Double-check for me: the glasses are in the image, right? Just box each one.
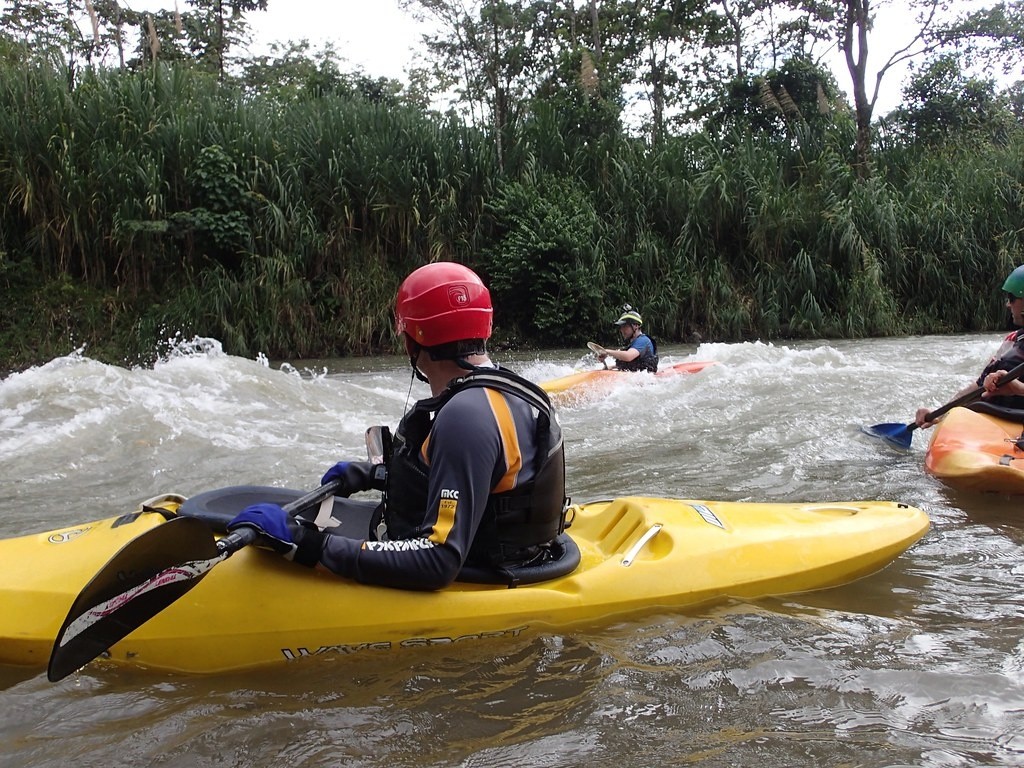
[1007,292,1024,303]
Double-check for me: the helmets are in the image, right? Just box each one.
[395,262,493,346]
[614,303,643,327]
[1001,265,1024,298]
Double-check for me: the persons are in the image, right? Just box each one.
[598,311,660,376]
[223,262,570,592]
[915,263,1024,429]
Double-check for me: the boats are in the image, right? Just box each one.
[538,362,716,397]
[926,381,1024,497]
[0,496,934,673]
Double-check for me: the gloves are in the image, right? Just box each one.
[321,461,377,499]
[226,502,321,567]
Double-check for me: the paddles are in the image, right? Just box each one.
[870,359,1023,448]
[45,457,375,685]
[586,341,609,369]
